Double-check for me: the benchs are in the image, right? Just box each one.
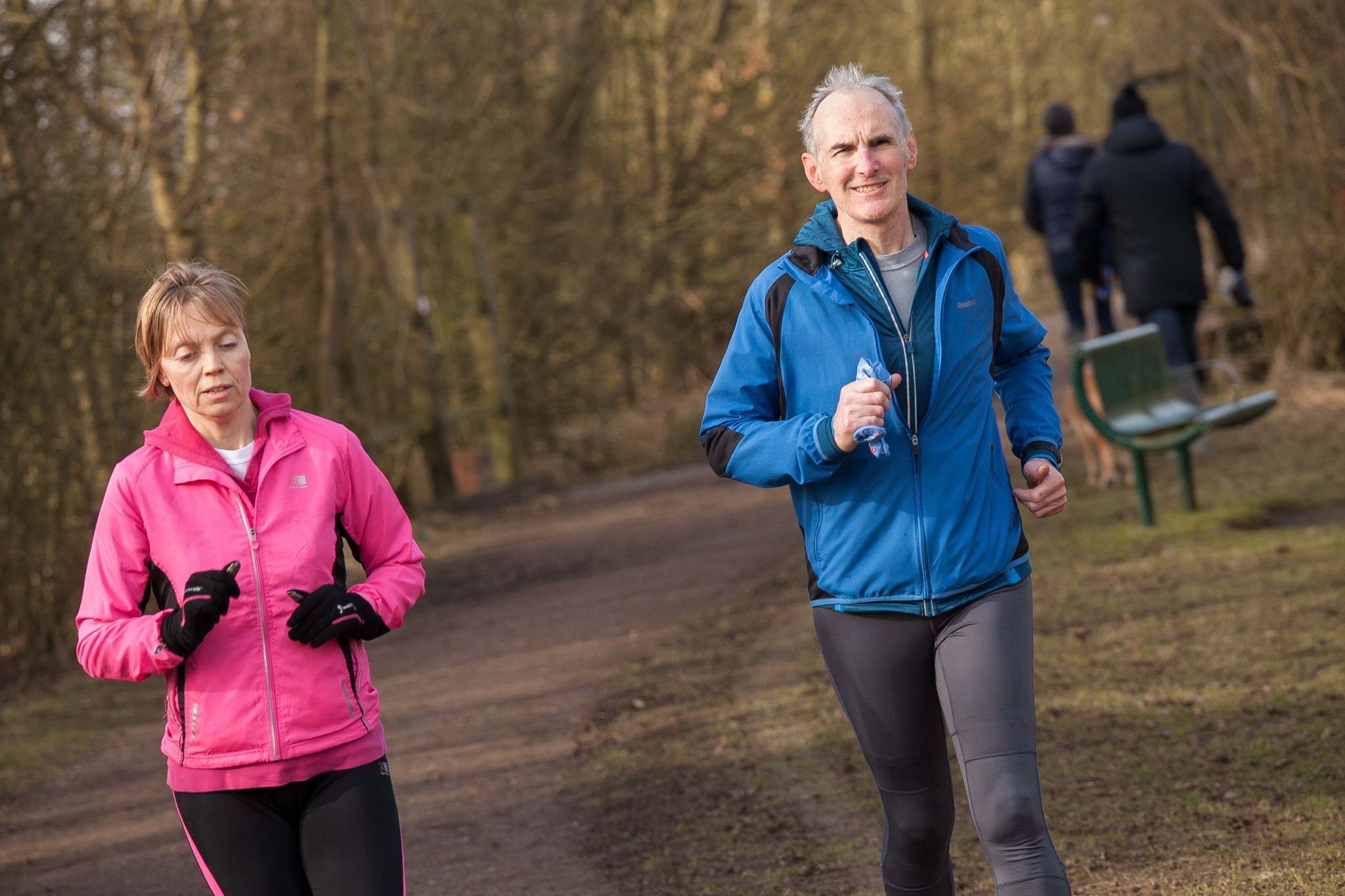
[1068,323,1278,530]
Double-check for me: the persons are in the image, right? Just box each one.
[1020,106,1117,353]
[72,267,429,896]
[698,63,1076,896]
[1085,84,1252,398]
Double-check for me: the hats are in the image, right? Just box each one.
[1114,84,1149,123]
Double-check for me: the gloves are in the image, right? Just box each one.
[168,568,240,652]
[284,584,391,649]
